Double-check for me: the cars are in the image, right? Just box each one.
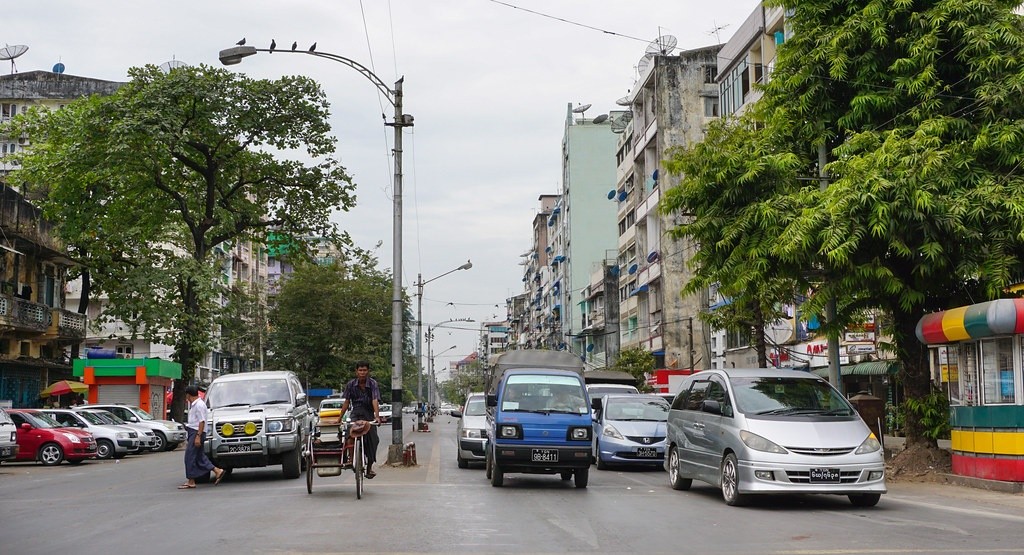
[661,366,889,508]
[590,393,673,472]
[378,402,392,425]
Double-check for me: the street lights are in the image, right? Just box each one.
[218,45,414,468]
[414,260,476,432]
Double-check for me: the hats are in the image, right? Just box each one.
[349,420,371,438]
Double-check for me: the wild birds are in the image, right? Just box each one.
[292,41,297,50]
[309,42,317,52]
[270,39,276,53]
[235,37,246,46]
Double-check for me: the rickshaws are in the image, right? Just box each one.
[303,406,380,500]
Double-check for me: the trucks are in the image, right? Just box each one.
[483,348,602,488]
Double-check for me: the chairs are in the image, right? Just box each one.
[521,384,552,409]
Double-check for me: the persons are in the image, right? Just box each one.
[431,404,436,416]
[459,405,463,415]
[546,385,585,412]
[79,393,88,405]
[178,385,226,489]
[44,397,52,408]
[507,384,527,410]
[422,402,426,418]
[329,361,382,480]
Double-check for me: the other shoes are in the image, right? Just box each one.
[366,471,376,479]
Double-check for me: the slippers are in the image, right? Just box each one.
[178,485,197,489]
[214,470,226,486]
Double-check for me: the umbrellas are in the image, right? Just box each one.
[39,380,89,397]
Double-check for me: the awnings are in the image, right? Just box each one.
[810,361,895,378]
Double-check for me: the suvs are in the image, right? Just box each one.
[200,369,315,480]
[0,407,21,466]
[70,401,188,453]
[4,408,100,466]
[34,407,158,460]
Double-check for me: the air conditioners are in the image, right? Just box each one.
[115,353,133,359]
[17,137,30,146]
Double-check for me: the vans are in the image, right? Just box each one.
[313,398,351,430]
[450,391,491,469]
[581,383,640,426]
